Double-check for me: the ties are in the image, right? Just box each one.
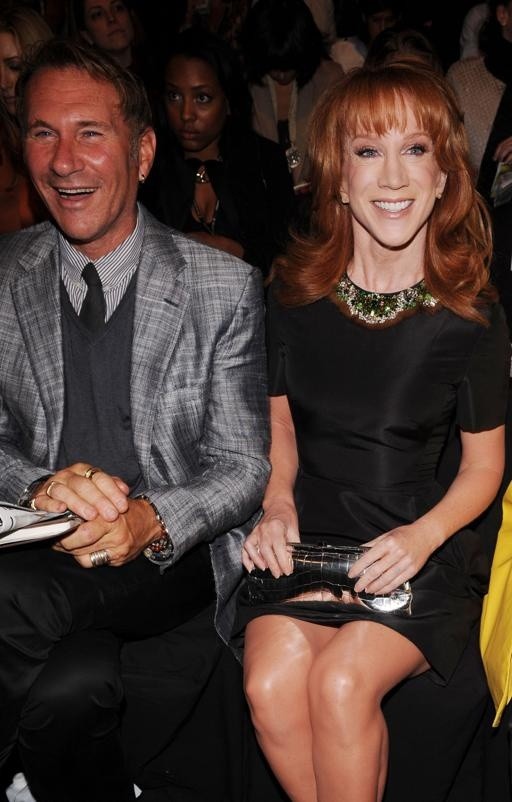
[78,262,106,336]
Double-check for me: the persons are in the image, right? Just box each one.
[0,30,276,800]
[229,49,509,800]
[0,0,512,323]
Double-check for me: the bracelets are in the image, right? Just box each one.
[134,493,170,553]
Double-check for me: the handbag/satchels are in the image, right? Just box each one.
[245,542,414,628]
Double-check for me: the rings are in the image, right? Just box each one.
[83,466,105,480]
[90,548,111,568]
[46,481,57,496]
[31,498,37,511]
